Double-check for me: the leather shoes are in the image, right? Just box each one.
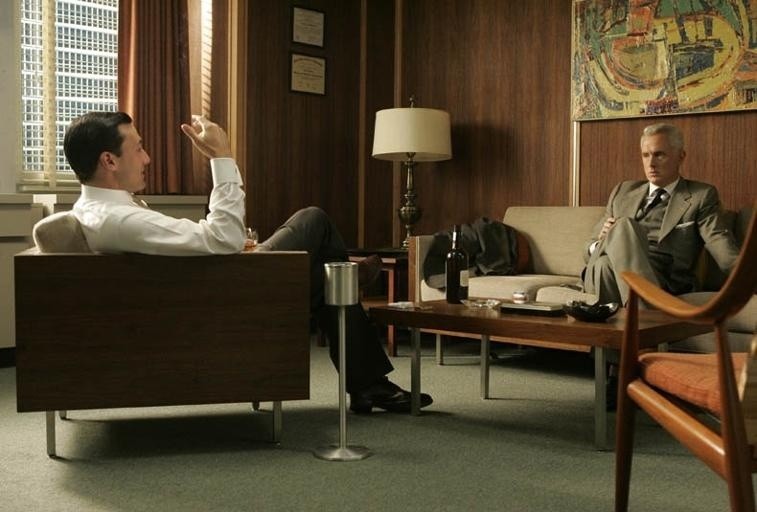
[350,385,433,413]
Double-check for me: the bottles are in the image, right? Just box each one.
[446,225,469,303]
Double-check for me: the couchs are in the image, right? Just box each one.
[12,210,311,457]
[405,205,757,366]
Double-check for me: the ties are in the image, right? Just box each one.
[635,189,666,220]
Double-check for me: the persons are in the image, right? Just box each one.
[65,111,434,415]
[582,124,744,405]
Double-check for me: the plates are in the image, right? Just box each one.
[460,298,501,310]
[562,301,619,322]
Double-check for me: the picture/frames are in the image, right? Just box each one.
[290,52,327,95]
[571,0,757,122]
[292,5,326,48]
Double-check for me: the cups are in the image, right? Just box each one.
[512,291,529,305]
[245,227,257,252]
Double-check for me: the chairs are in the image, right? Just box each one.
[611,208,756,512]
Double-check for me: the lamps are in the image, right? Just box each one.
[372,93,452,247]
